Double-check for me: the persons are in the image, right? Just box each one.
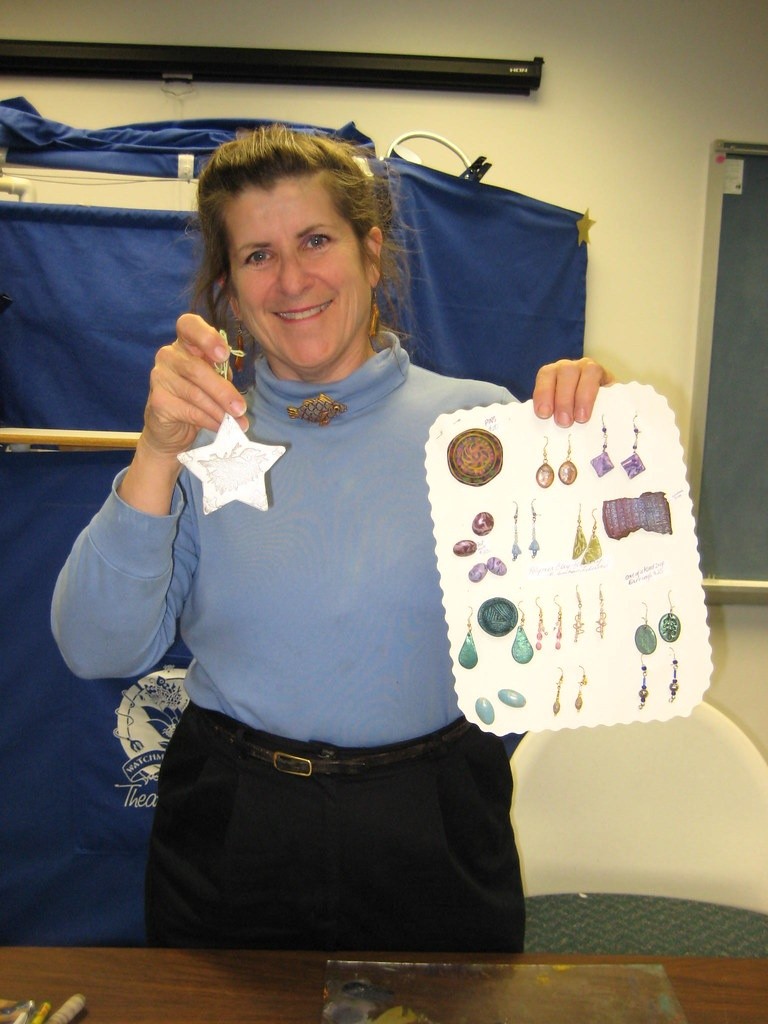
[52,128,608,961]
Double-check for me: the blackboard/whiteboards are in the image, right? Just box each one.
[687,138,768,608]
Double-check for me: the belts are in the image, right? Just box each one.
[192,707,472,777]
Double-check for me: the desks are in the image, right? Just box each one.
[1,946,768,1023]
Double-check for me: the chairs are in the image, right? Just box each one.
[508,701,768,958]
